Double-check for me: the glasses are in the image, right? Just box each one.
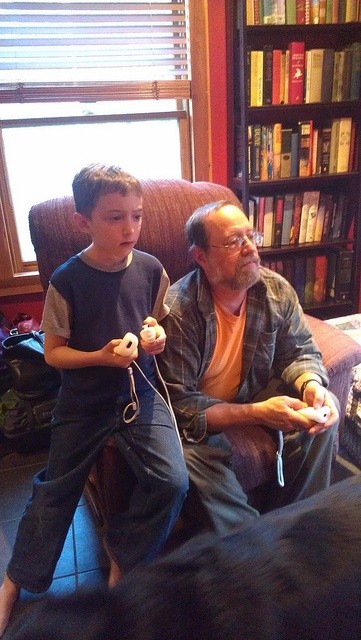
[198,233,264,254]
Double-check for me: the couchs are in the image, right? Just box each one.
[325,309,361,473]
[27,178,360,549]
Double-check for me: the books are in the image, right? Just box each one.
[250,190,359,252]
[244,38,361,115]
[248,118,361,190]
[261,254,353,309]
[248,0,361,28]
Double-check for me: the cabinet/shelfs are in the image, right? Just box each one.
[227,0,361,322]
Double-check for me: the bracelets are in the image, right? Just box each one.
[299,379,320,402]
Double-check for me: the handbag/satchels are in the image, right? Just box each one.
[1,312,40,336]
[0,329,61,391]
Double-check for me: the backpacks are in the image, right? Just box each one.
[0,388,60,453]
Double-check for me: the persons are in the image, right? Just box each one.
[160,199,343,529]
[0,165,190,640]
